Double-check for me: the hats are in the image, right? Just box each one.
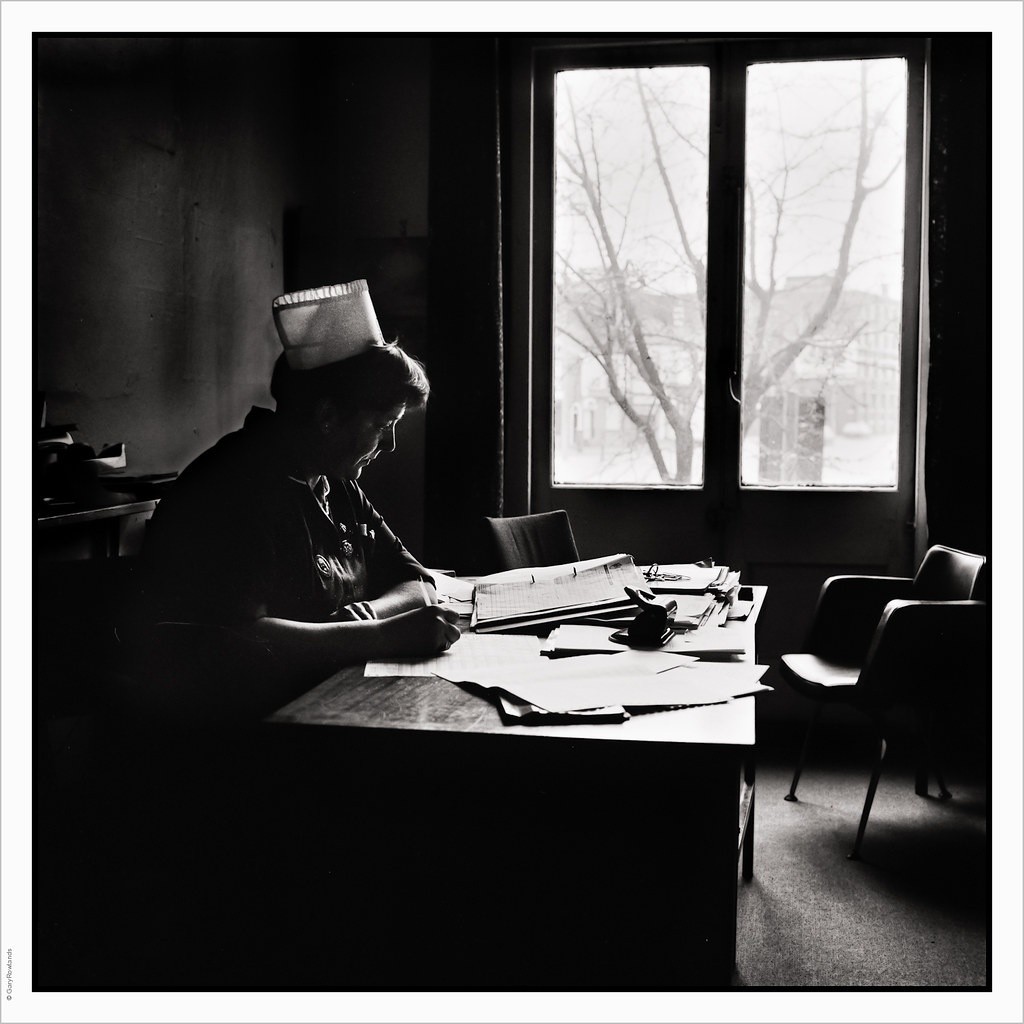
[271,278,384,371]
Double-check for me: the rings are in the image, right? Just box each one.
[444,641,450,649]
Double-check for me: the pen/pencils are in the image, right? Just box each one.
[415,572,431,606]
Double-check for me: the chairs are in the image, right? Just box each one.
[480,509,581,575]
[33,501,277,949]
[779,544,992,861]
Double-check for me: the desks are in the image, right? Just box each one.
[263,586,758,993]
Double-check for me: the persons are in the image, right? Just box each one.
[108,278,459,720]
